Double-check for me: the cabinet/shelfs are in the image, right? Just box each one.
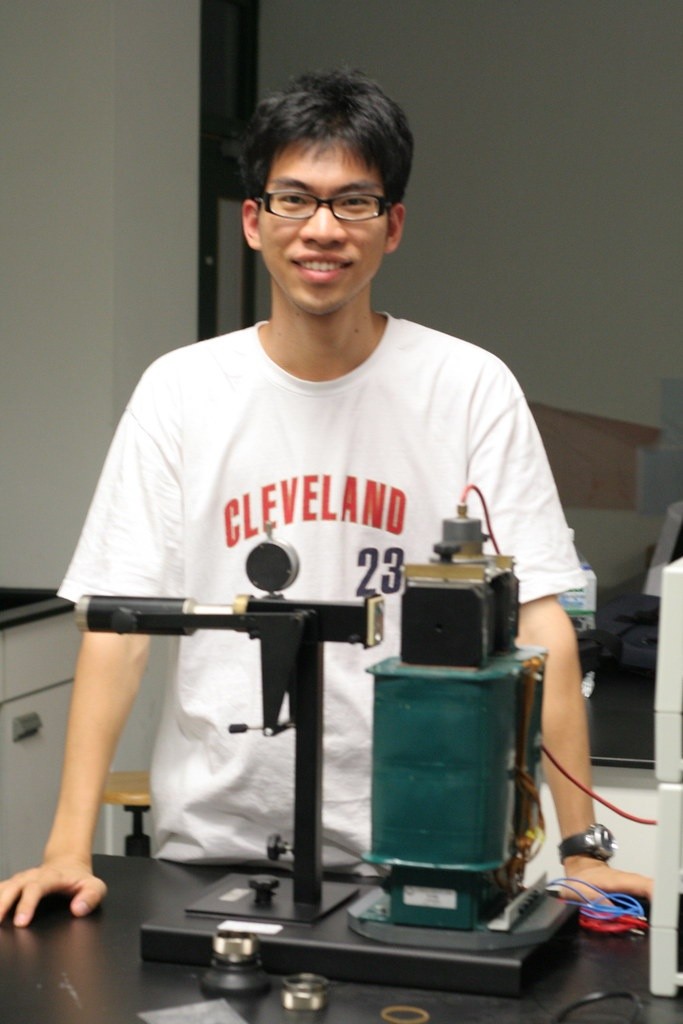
[0,612,116,879]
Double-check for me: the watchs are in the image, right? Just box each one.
[559,822,616,866]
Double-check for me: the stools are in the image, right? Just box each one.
[101,770,153,858]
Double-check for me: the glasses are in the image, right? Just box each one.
[261,191,387,222]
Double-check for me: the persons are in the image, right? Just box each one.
[0,72,654,927]
